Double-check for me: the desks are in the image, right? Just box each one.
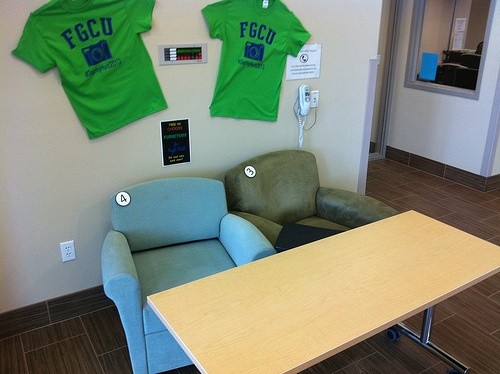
[146,209,500,374]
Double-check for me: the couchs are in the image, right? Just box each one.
[100,177,277,374]
[224,150,400,253]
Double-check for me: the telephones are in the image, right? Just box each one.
[296,85,311,150]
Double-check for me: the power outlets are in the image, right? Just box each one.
[310,90,319,108]
[59,240,76,263]
